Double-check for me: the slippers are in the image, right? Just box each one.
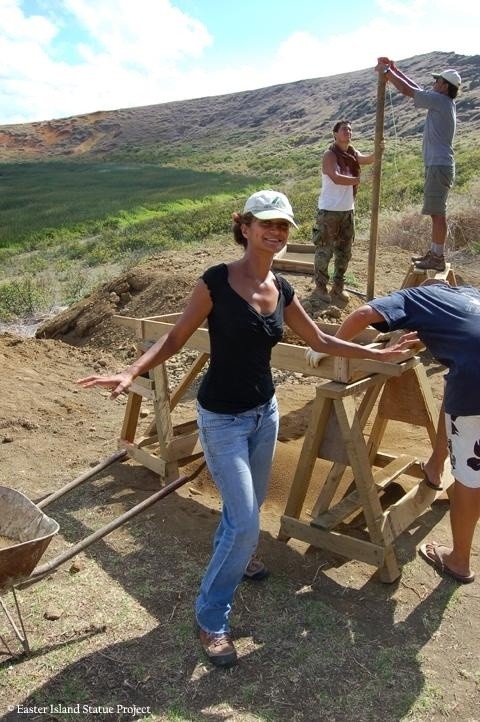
[420,543,475,582]
[419,460,443,491]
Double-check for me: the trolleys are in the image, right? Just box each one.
[0,448,190,661]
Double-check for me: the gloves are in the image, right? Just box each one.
[303,347,330,368]
[373,330,404,349]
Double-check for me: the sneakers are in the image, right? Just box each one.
[199,625,237,668]
[411,249,430,263]
[244,555,267,579]
[414,251,446,271]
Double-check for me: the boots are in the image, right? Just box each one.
[331,278,349,302]
[313,279,332,302]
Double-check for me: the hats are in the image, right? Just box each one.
[431,68,462,90]
[242,189,300,231]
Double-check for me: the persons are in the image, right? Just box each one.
[304,278,480,583]
[312,121,386,300]
[374,59,461,272]
[76,190,420,666]
